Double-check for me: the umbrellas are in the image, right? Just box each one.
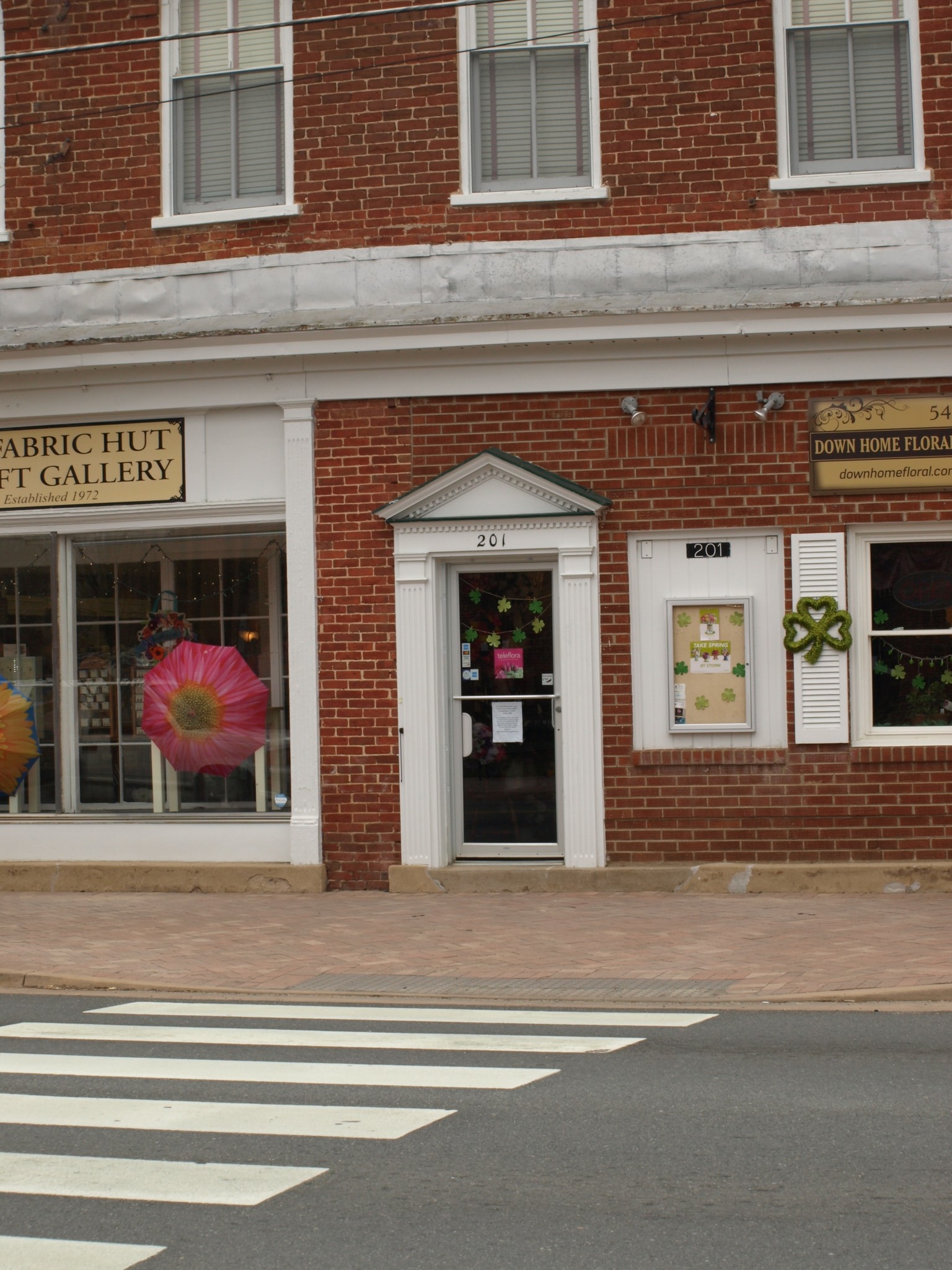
[140,639,269,778]
[0,677,43,798]
